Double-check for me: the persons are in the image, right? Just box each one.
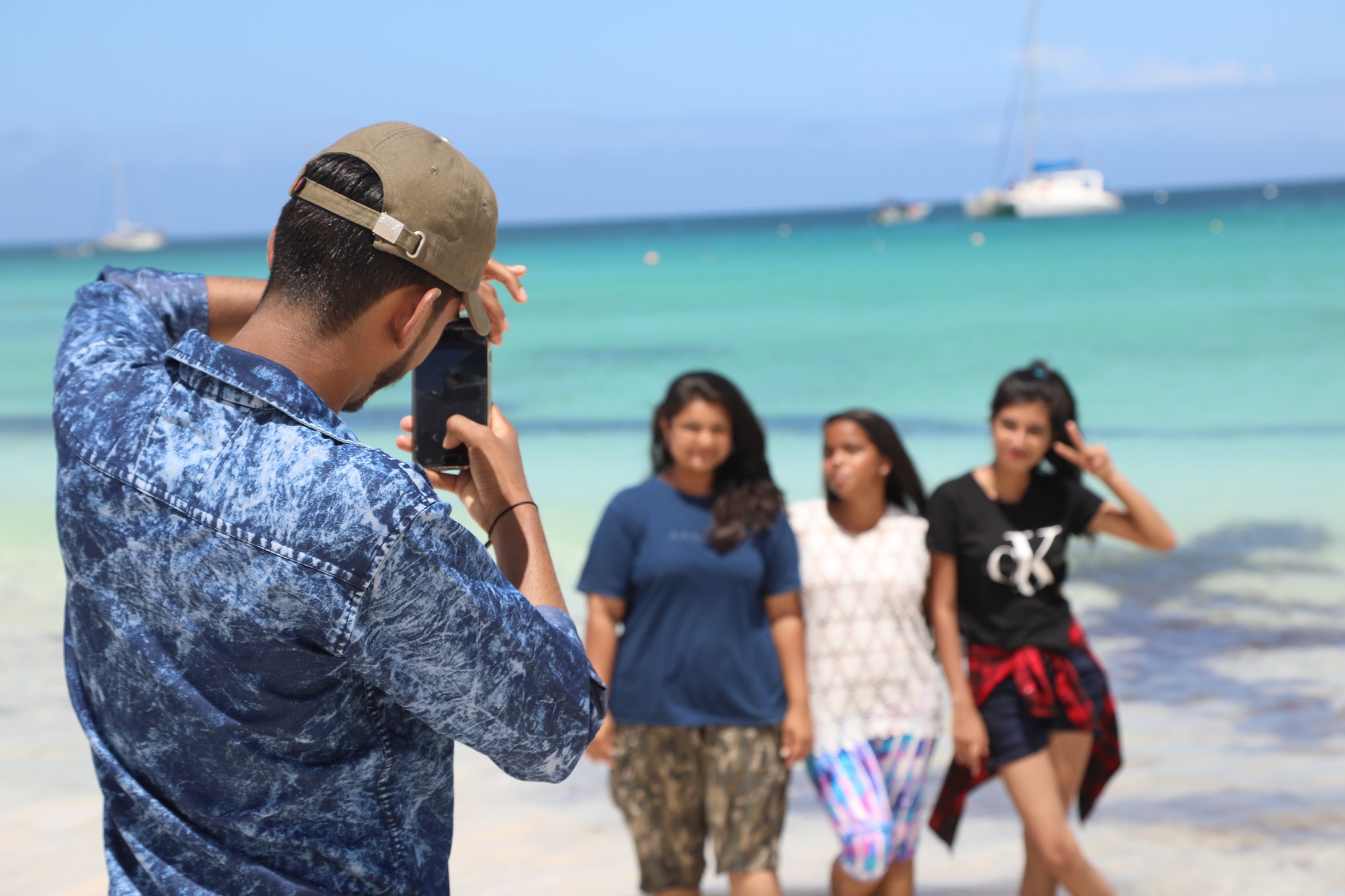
[777,407,959,896]
[919,359,1175,896]
[52,121,608,896]
[581,371,813,896]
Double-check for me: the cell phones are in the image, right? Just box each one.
[411,315,492,469]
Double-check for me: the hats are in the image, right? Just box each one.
[289,120,495,336]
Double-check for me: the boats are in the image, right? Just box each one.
[877,201,935,226]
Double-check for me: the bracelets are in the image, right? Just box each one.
[482,500,538,550]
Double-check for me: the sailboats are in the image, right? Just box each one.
[98,157,164,253]
[964,0,1124,219]
